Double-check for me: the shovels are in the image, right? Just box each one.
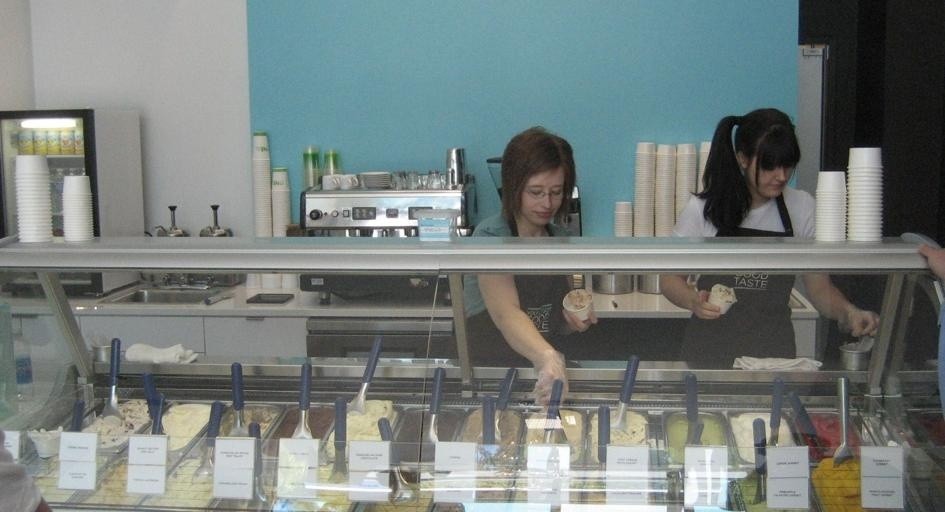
[192,401,225,477]
[480,364,520,440]
[227,360,249,437]
[338,331,387,411]
[329,396,349,481]
[832,376,855,464]
[416,366,444,442]
[375,418,418,498]
[101,338,121,415]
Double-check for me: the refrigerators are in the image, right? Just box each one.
[0,107,146,302]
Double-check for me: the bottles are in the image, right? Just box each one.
[15,122,83,155]
[447,149,464,191]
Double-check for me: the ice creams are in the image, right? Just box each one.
[710,284,737,303]
[565,288,590,311]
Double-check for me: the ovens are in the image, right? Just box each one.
[303,317,456,360]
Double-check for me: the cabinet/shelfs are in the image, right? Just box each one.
[75,318,306,363]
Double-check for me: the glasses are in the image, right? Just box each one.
[522,189,566,198]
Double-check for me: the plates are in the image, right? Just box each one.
[359,173,392,190]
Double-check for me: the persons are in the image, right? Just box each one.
[660,107,879,367]
[921,241,945,417]
[440,125,599,407]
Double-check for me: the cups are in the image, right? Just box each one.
[562,291,595,322]
[248,134,298,295]
[14,153,94,243]
[816,147,883,244]
[839,340,870,373]
[614,143,710,237]
[304,144,358,191]
[399,171,446,189]
[709,283,738,314]
